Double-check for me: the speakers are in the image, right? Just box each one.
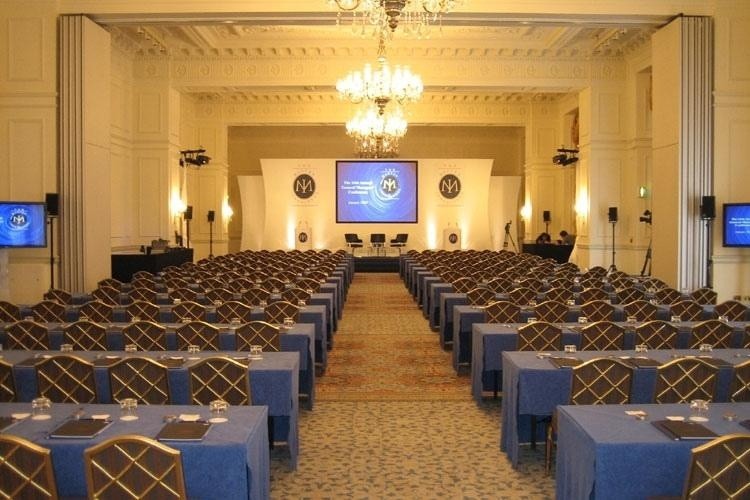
[544,211,549,221]
[208,211,214,221]
[609,207,617,223]
[702,196,715,220]
[46,193,60,217]
[184,206,192,219]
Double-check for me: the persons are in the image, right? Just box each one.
[559,230,578,245]
[536,232,551,243]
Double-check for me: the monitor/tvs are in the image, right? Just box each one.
[0,202,48,248]
[723,203,750,247]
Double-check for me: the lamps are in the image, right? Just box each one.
[327,1,466,162]
[549,147,579,167]
[178,148,212,168]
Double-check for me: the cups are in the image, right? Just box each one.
[32,396,51,412]
[627,316,636,325]
[635,344,648,353]
[528,300,536,306]
[527,317,537,323]
[209,400,227,413]
[700,343,712,353]
[564,344,577,354]
[250,344,262,357]
[284,317,296,327]
[567,299,575,306]
[214,299,222,306]
[690,399,709,415]
[671,315,681,323]
[173,298,182,304]
[259,299,267,307]
[718,315,729,322]
[125,344,137,356]
[297,299,306,306]
[120,398,137,411]
[578,316,588,324]
[230,318,241,327]
[60,344,73,352]
[649,299,658,306]
[187,345,199,357]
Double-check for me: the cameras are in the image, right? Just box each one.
[640,210,651,225]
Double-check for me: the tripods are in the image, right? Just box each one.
[503,230,518,254]
[607,252,617,273]
[640,239,651,276]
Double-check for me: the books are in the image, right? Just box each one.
[155,355,183,370]
[15,353,53,371]
[155,416,214,444]
[82,354,121,368]
[230,355,254,369]
[48,418,116,439]
[1,416,26,433]
[503,320,749,443]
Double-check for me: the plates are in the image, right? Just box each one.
[178,414,203,424]
[120,416,138,421]
[32,414,52,421]
[91,413,112,419]
[689,416,710,423]
[248,354,264,361]
[666,415,684,421]
[207,417,228,423]
[12,412,31,419]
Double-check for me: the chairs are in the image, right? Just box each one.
[344,233,364,258]
[370,233,386,257]
[390,233,409,256]
